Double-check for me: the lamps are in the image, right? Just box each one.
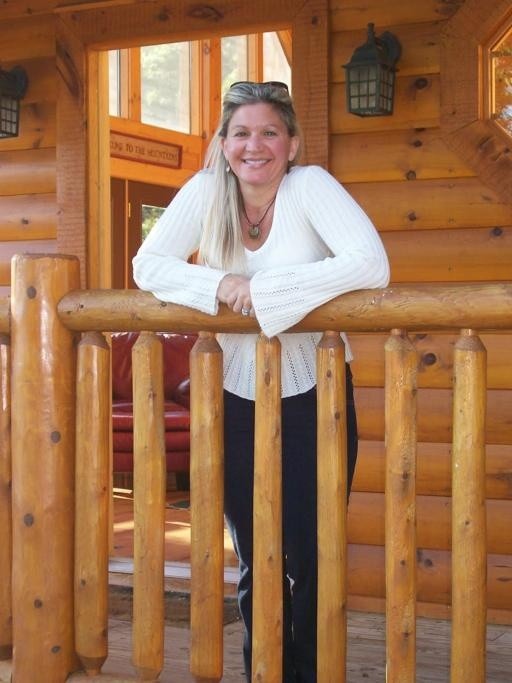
[339,20,403,120]
[1,63,31,140]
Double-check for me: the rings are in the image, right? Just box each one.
[241,306,250,314]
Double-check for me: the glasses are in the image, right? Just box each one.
[230,81,289,92]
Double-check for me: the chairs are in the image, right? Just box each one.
[110,331,199,473]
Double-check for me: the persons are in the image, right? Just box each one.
[132,79,393,682]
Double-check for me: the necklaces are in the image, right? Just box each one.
[237,187,276,239]
